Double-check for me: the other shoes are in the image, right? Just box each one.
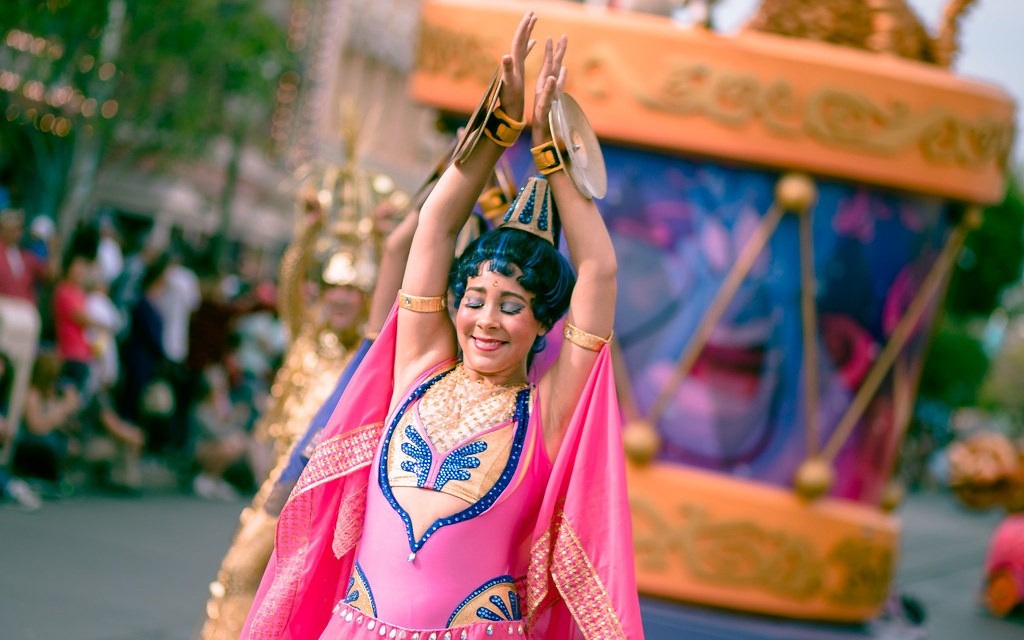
[5,478,40,512]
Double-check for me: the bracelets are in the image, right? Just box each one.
[486,106,527,148]
[529,139,563,175]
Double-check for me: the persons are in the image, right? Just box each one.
[306,8,618,640]
[0,126,520,514]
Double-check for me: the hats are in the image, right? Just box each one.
[292,91,381,318]
[498,174,562,251]
[454,213,488,258]
